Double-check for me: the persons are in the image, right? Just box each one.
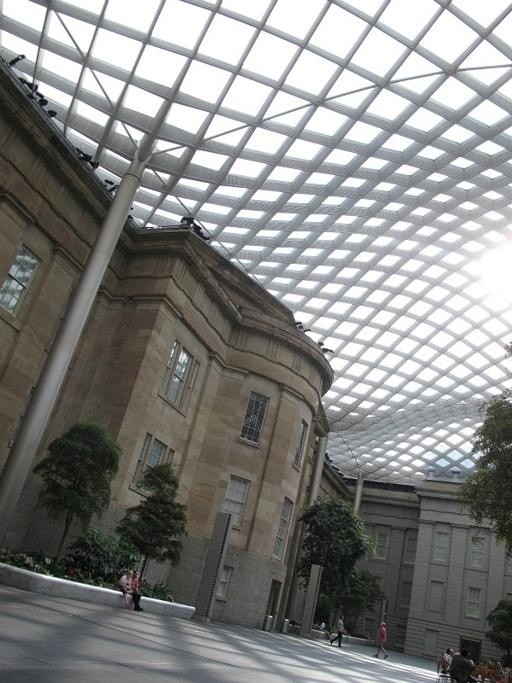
[118,570,133,606]
[373,620,390,659]
[328,615,348,649]
[319,620,327,629]
[427,647,511,682]
[132,569,145,611]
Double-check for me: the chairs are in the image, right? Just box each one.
[437,652,512,683]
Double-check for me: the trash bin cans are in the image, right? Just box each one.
[263,615,274,631]
[280,618,290,633]
